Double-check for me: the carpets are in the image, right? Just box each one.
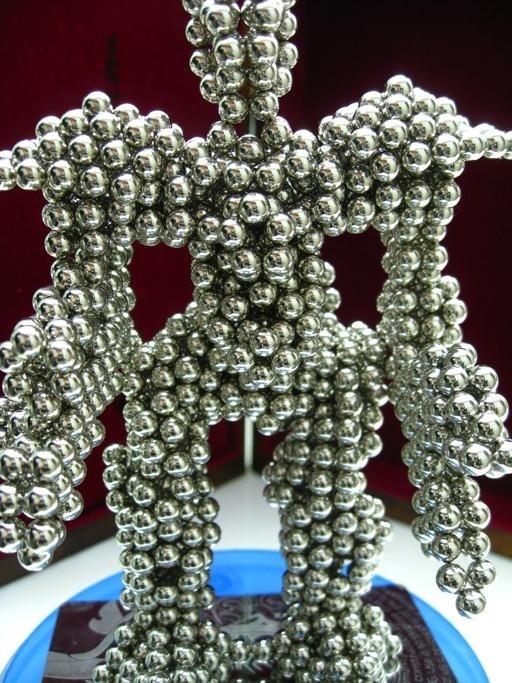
[39,582,459,682]
